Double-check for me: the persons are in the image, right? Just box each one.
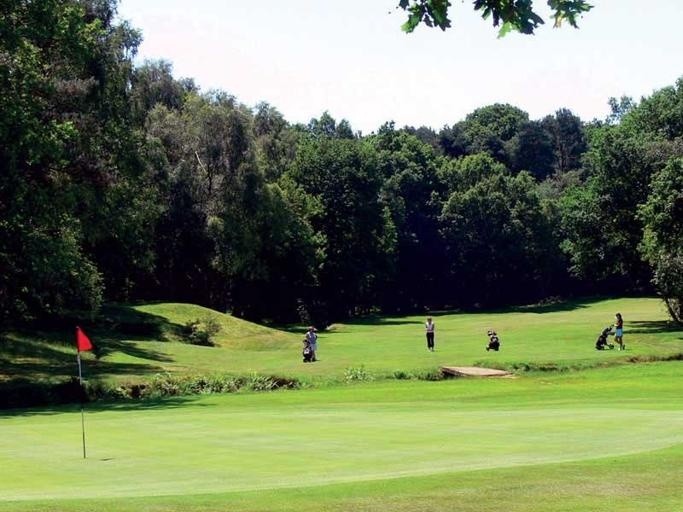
[302,338,315,362]
[614,312,625,351]
[486,334,500,352]
[306,326,318,360]
[425,316,435,351]
